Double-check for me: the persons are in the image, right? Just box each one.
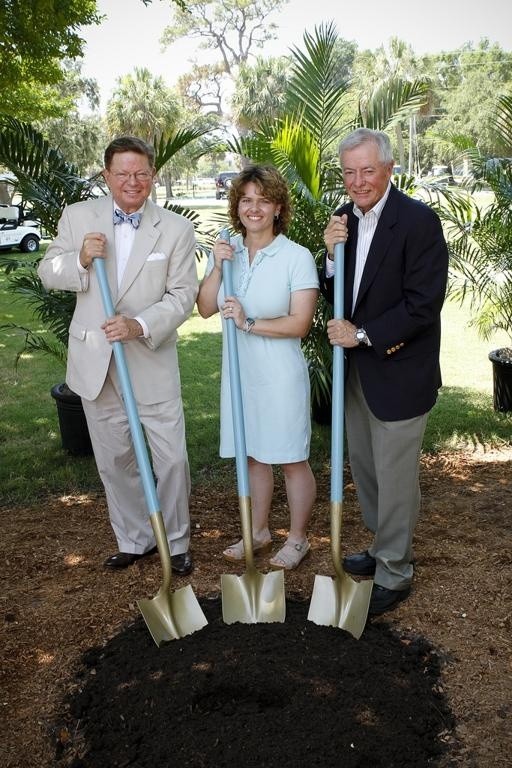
[37,137,200,577]
[319,127,448,617]
[196,162,320,570]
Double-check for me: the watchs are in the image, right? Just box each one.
[354,329,367,344]
[243,318,255,333]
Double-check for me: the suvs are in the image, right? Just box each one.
[215,171,242,200]
[392,166,404,183]
[430,166,451,185]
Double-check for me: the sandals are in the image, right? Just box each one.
[222,537,311,572]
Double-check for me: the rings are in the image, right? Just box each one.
[229,308,232,313]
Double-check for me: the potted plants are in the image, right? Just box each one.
[188,19,482,431]
[0,65,232,455]
[413,91,512,416]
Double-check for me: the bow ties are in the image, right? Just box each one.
[113,209,141,228]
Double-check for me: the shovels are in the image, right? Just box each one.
[92,238,212,653]
[219,228,289,623]
[305,211,376,641]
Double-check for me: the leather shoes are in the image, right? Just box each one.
[104,545,193,575]
[344,551,409,615]
